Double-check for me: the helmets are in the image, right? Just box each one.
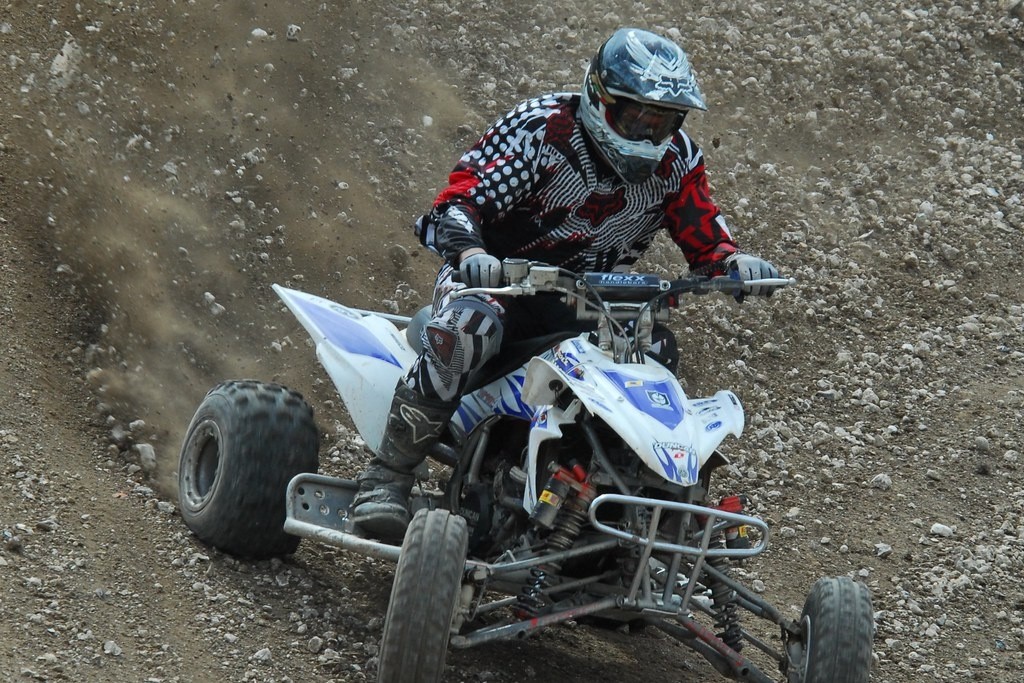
[579,28,707,184]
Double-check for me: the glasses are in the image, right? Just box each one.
[590,36,689,146]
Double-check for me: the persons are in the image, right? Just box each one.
[352,26,778,538]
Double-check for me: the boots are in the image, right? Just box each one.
[349,375,462,545]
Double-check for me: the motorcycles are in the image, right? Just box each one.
[176,256,883,683]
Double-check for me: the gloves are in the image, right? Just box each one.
[459,251,502,288]
[723,252,778,304]
